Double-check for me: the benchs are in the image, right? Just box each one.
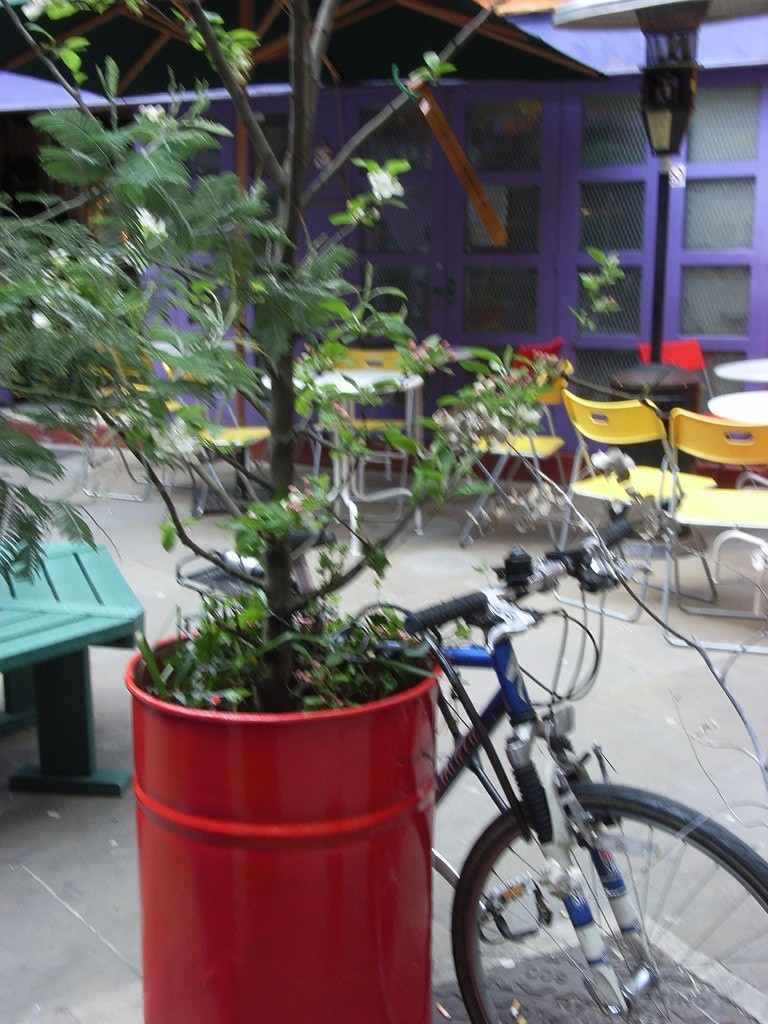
[0,541,147,799]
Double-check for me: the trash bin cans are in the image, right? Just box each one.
[611,361,701,543]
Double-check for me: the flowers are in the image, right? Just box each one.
[0,1,627,708]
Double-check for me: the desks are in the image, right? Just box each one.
[261,370,426,554]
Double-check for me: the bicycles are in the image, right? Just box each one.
[170,496,767,1024]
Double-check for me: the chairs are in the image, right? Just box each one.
[159,358,270,508]
[456,337,768,659]
[313,336,424,477]
[83,345,182,499]
